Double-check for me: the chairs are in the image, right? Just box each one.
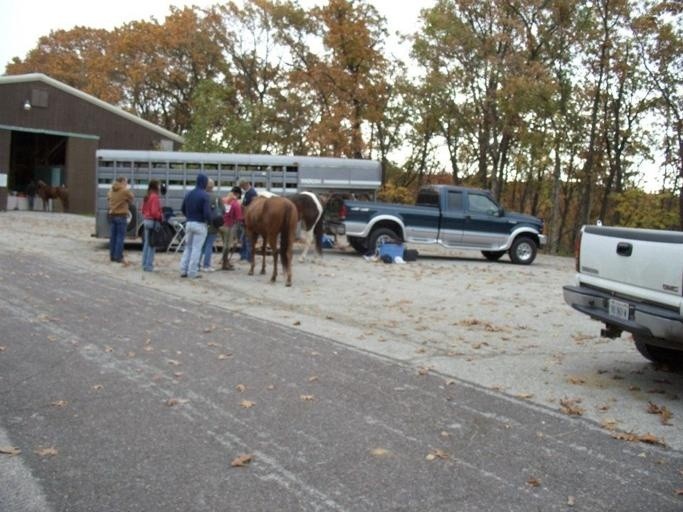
[167,217,186,255]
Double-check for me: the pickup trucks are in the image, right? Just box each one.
[562,217,682,358]
[339,183,549,266]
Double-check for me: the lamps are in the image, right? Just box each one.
[24,100,31,111]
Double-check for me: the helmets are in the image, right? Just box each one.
[231,187,241,199]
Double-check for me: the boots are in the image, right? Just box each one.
[222,252,235,270]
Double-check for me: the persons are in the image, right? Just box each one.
[58,182,71,212]
[138,177,165,271]
[24,178,37,211]
[105,175,136,267]
[213,185,245,271]
[235,176,260,266]
[178,174,214,280]
[194,178,227,274]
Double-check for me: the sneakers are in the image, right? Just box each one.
[180,265,214,280]
[141,262,160,273]
[111,255,130,266]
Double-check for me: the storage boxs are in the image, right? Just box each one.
[380,243,404,260]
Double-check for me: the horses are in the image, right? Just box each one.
[243,191,327,287]
[35,179,70,213]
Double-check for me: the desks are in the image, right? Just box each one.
[7,196,52,211]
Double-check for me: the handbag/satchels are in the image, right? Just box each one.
[212,207,224,228]
[149,228,168,247]
